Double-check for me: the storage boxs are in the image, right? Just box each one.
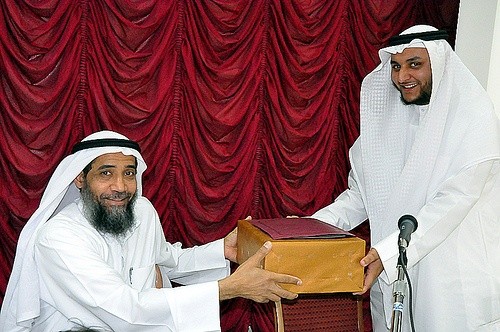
[235,220,366,294]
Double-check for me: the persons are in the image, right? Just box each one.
[286,25,500,332]
[0,131,302,332]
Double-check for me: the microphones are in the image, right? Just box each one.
[398,215,418,251]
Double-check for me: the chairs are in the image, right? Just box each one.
[275,295,364,332]
